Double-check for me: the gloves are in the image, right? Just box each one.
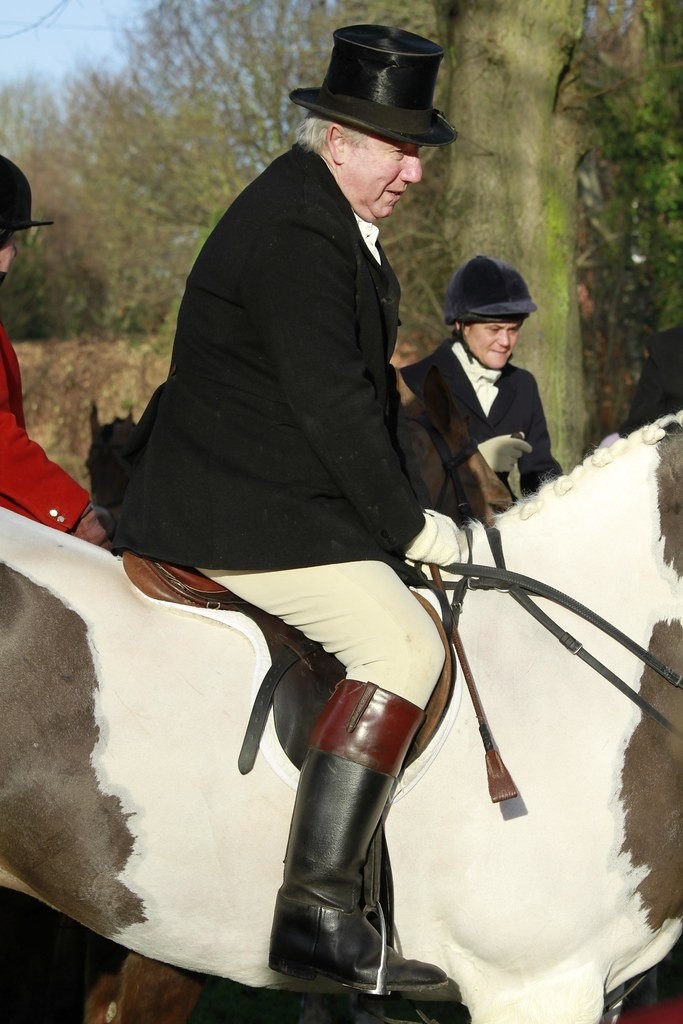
[402,507,461,583]
[477,432,534,472]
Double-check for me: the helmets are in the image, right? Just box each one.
[445,255,537,327]
[0,155,54,229]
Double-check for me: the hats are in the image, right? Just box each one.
[288,24,458,147]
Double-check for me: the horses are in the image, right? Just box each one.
[0,400,683,1024]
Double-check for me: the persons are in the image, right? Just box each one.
[401,256,563,514]
[617,326,683,438]
[0,155,111,551]
[109,24,469,992]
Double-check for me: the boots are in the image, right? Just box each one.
[269,679,450,991]
